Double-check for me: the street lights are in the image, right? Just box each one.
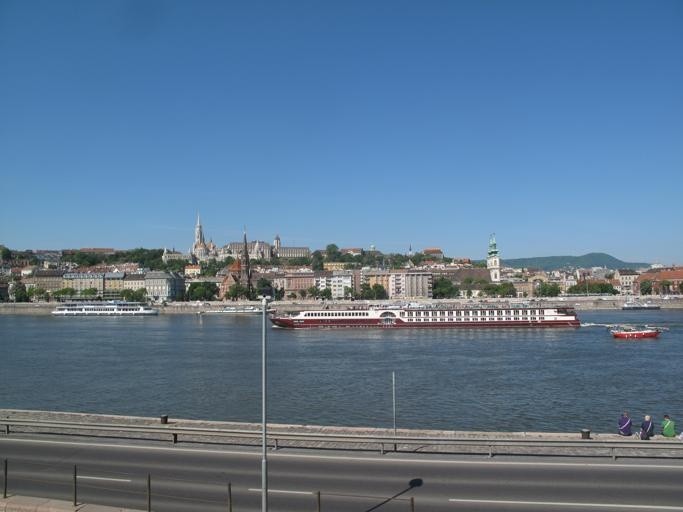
[256,295,272,512]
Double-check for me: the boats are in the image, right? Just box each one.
[620,295,662,312]
[196,305,279,315]
[609,325,663,338]
[49,300,159,316]
[267,305,581,328]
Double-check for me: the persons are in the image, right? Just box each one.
[660,415,676,437]
[640,416,654,436]
[615,412,632,436]
